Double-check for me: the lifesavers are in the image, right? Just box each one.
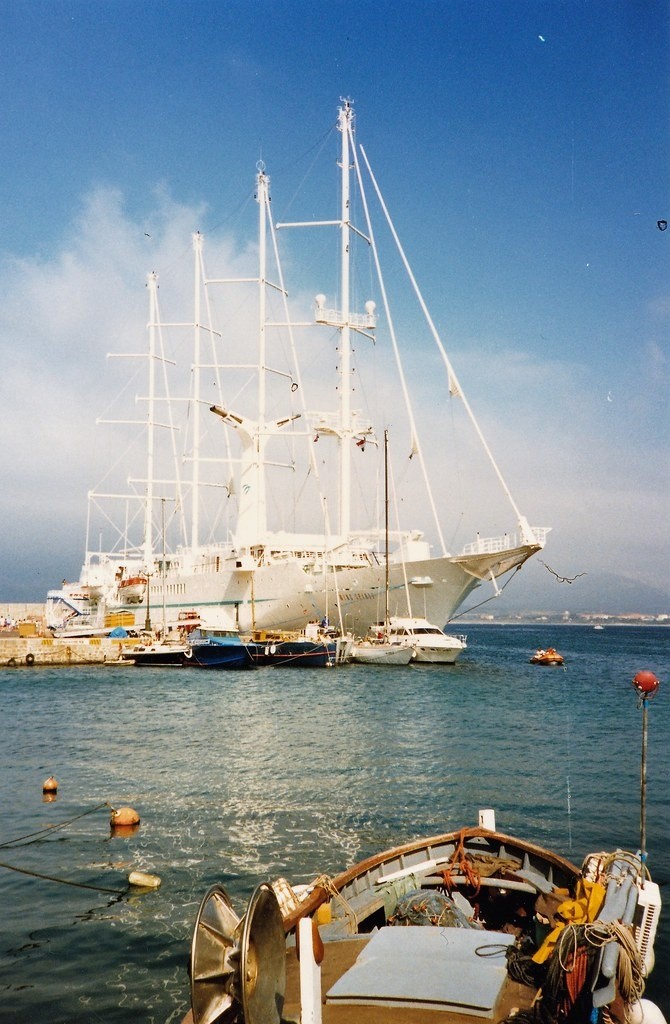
[26,654,34,665]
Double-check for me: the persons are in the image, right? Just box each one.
[534,646,555,659]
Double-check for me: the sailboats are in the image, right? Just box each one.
[45,96,552,636]
[349,429,417,665]
[318,496,357,665]
[119,499,189,667]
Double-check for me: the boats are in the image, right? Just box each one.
[593,624,604,629]
[183,627,261,670]
[370,616,469,665]
[250,620,337,669]
[530,654,564,666]
